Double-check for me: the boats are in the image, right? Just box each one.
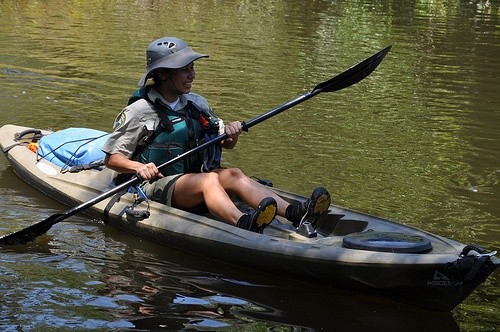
[0,122,499,314]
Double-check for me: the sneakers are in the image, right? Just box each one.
[242,197,278,234]
[292,187,331,228]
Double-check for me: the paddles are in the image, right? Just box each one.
[0,45,392,245]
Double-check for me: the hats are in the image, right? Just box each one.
[138,37,210,86]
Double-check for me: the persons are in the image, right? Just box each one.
[101,36,332,234]
